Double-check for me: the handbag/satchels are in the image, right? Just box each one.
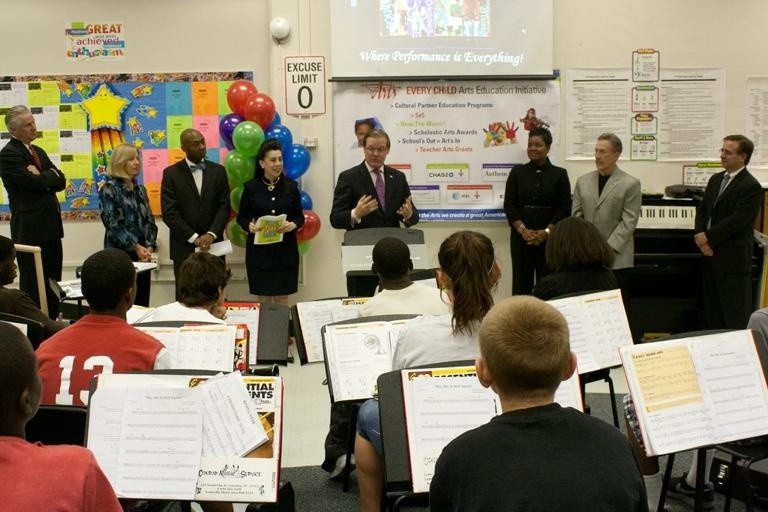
[666,185,703,198]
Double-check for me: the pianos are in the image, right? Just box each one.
[624,192,706,332]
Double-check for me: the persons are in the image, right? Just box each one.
[482,121,520,148]
[503,125,573,297]
[322,236,448,481]
[0,105,67,321]
[328,127,420,298]
[663,309,768,510]
[236,138,306,313]
[1,234,124,511]
[519,108,551,132]
[428,297,648,511]
[569,134,642,347]
[97,142,159,309]
[131,251,232,324]
[355,231,500,511]
[348,117,377,149]
[32,252,233,511]
[160,127,232,305]
[693,134,764,331]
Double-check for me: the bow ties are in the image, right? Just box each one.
[190,161,206,174]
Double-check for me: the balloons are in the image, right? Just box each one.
[218,80,322,255]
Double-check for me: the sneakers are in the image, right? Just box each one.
[667,471,714,508]
[329,454,356,482]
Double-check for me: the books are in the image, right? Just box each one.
[83,305,282,504]
[296,289,767,491]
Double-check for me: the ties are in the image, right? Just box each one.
[713,174,730,209]
[30,144,41,170]
[372,169,386,213]
